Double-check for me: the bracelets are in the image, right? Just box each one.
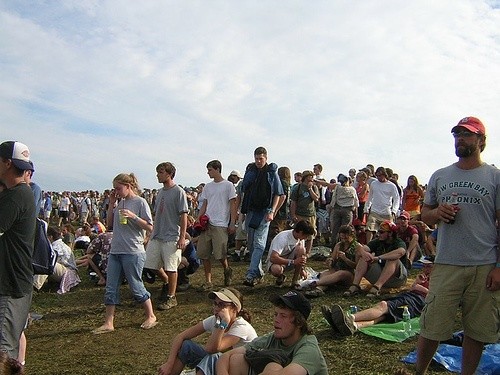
[288,259,292,267]
[218,321,228,330]
[378,256,382,263]
[270,209,275,213]
[133,216,138,221]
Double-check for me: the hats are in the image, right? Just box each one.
[302,171,316,175]
[359,168,371,173]
[0,140,35,172]
[230,170,243,179]
[451,116,485,135]
[268,293,311,320]
[208,288,241,310]
[399,211,410,219]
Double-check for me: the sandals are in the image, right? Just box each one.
[365,285,381,298]
[343,284,362,297]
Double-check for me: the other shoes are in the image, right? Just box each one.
[294,281,317,291]
[331,304,357,336]
[292,284,302,291]
[244,278,264,287]
[157,282,178,310]
[197,281,214,293]
[175,281,192,291]
[224,266,234,286]
[275,274,286,288]
[305,286,325,298]
[321,305,335,330]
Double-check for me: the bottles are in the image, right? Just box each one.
[177,239,190,248]
[402,307,412,332]
[444,193,458,225]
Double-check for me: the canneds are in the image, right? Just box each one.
[119,209,127,224]
[350,305,358,314]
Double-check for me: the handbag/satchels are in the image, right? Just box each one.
[245,349,292,373]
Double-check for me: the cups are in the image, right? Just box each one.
[118,209,127,225]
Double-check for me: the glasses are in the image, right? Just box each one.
[453,129,477,137]
[378,230,390,234]
[213,301,234,309]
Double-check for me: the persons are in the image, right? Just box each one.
[0,141,439,375]
[413,117,500,375]
